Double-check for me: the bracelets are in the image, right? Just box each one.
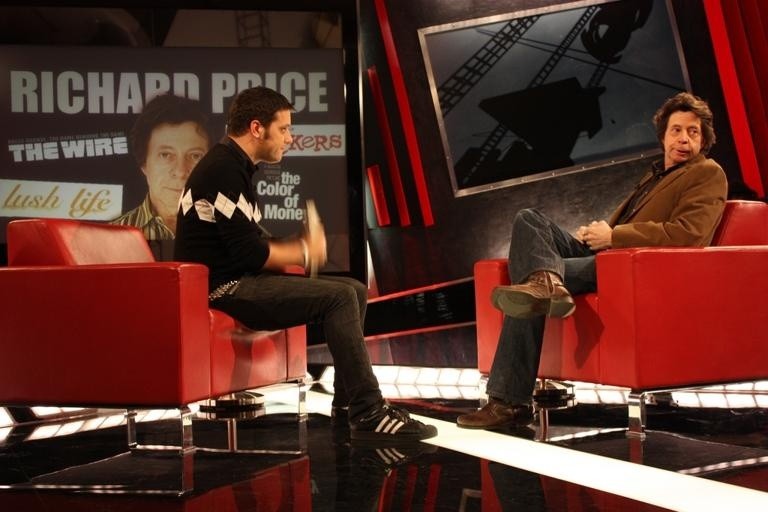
[300,238,309,269]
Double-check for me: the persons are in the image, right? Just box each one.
[173,87,437,442]
[332,442,438,512]
[457,93,728,428]
[108,90,219,261]
[474,428,546,512]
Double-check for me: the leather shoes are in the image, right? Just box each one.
[457,395,536,428]
[490,271,577,319]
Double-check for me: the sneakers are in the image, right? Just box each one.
[331,398,437,442]
[351,445,423,477]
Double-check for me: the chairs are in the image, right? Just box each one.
[0,218,308,454]
[1,449,314,512]
[474,200,767,438]
[478,437,768,511]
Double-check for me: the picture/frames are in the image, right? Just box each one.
[416,0,693,199]
